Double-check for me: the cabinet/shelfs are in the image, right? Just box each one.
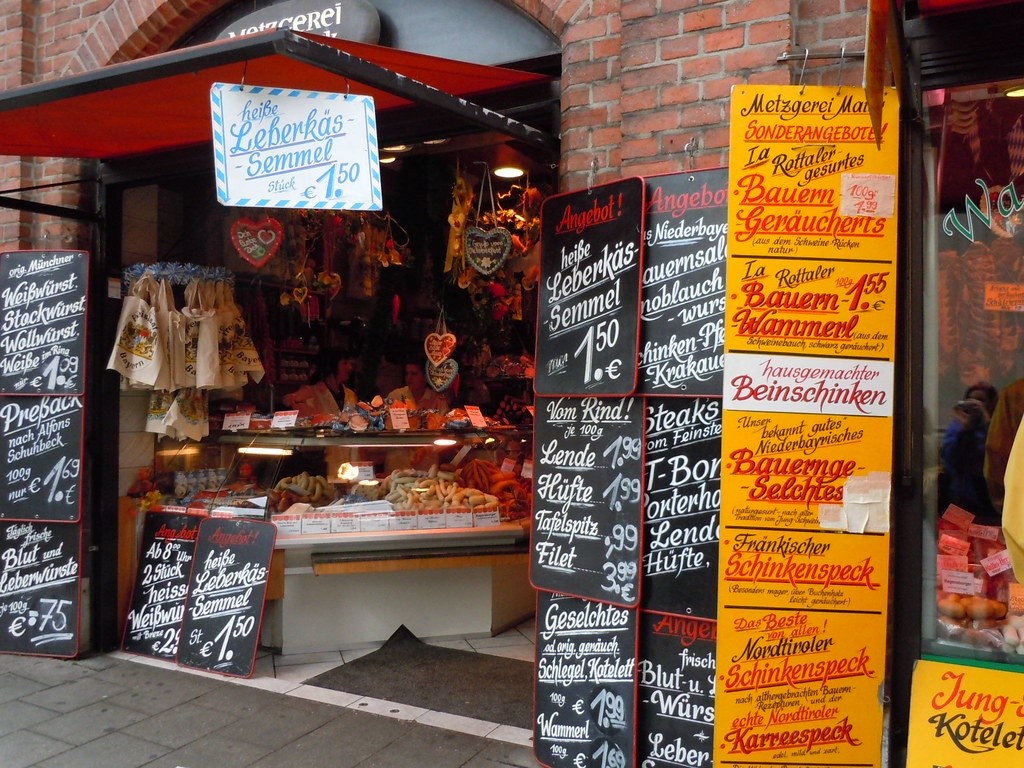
[131,425,535,654]
[197,152,556,424]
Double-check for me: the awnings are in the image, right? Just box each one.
[0,27,562,158]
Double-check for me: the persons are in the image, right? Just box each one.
[385,360,448,472]
[935,380,1003,527]
[282,339,364,475]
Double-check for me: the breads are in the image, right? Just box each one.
[936,582,1024,656]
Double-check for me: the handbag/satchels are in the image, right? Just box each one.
[107,276,267,441]
